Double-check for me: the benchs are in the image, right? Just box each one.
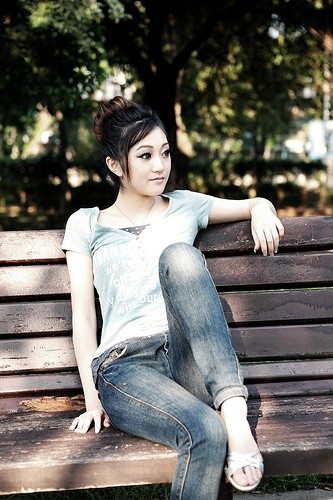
[0,214,333,495]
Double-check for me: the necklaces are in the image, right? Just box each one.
[110,196,157,226]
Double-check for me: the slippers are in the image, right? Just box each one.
[224,450,264,491]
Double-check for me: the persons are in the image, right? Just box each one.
[58,95,286,500]
